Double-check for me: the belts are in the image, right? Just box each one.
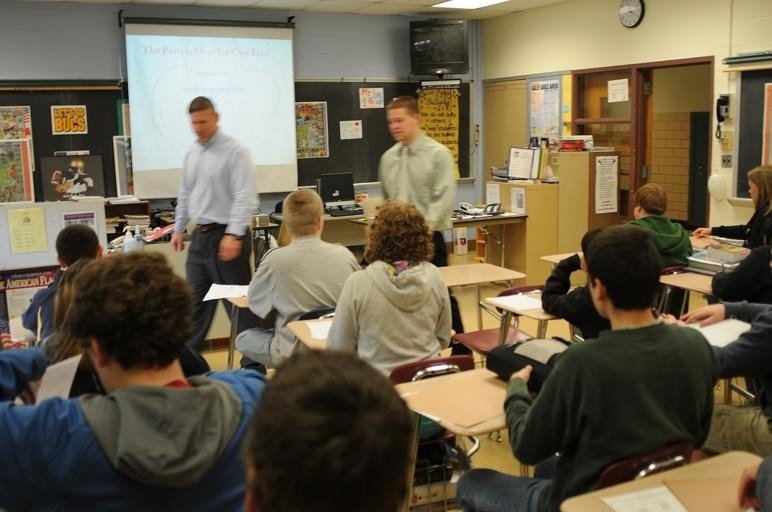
[195,223,227,232]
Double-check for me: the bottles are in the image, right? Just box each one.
[543,141,560,184]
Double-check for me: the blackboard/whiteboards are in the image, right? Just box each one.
[294,75,477,189]
[0,86,125,207]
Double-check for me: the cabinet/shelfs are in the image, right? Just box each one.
[105,197,154,249]
[542,151,620,255]
[484,180,559,289]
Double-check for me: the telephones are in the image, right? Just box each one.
[715,93,734,127]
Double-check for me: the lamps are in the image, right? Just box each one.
[619,1,644,28]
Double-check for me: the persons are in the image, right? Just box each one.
[540,229,658,340]
[326,201,453,438]
[377,95,458,296]
[171,96,266,376]
[661,165,771,460]
[1,225,411,512]
[457,225,720,512]
[739,459,771,511]
[626,182,692,320]
[235,188,361,369]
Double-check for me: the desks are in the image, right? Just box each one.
[448,213,526,288]
[270,197,382,248]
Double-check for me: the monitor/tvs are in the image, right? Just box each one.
[408,18,471,76]
